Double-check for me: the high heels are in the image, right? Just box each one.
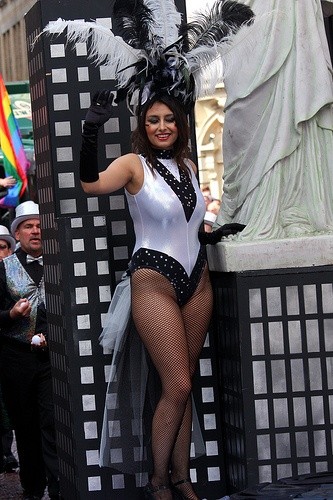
[147,477,172,500]
[169,478,198,500]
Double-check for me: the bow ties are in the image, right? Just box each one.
[26,254,44,266]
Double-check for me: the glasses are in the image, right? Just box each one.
[0,244,7,249]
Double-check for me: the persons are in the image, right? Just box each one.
[78,88,247,500]
[0,176,49,500]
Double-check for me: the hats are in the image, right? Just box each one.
[11,201,40,236]
[0,225,16,252]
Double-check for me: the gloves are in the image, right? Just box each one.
[197,222,247,246]
[80,89,115,182]
